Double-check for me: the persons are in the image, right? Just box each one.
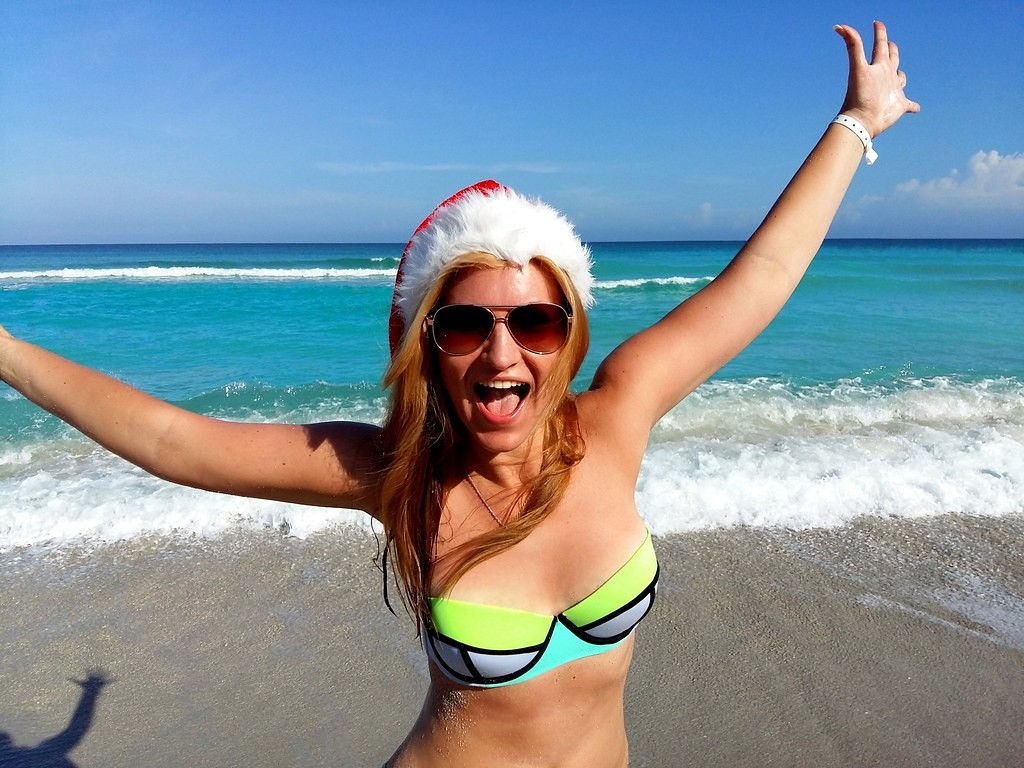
[0,18,923,768]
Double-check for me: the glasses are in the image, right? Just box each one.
[420,303,576,356]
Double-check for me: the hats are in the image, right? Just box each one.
[389,179,596,351]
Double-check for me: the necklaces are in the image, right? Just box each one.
[452,449,549,530]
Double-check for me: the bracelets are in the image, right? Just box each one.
[829,115,879,166]
